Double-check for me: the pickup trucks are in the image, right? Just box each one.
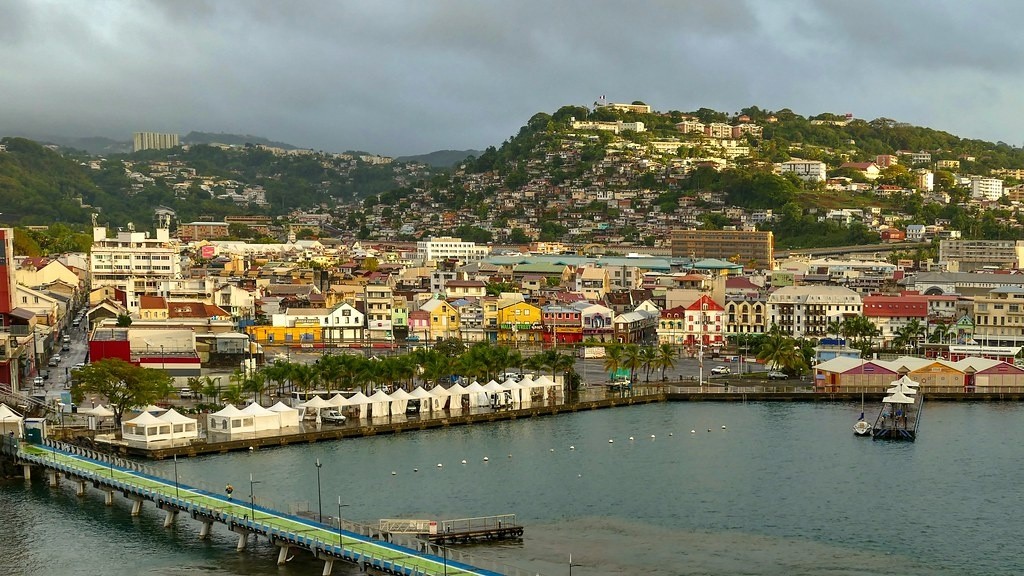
[711,366,730,374]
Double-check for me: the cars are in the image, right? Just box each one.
[48,357,58,367]
[33,377,44,386]
[53,354,61,361]
[62,343,69,351]
[72,307,87,327]
[385,335,395,341]
[40,370,50,379]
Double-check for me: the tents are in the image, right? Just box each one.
[122,409,198,441]
[207,401,299,434]
[85,404,115,428]
[300,376,556,418]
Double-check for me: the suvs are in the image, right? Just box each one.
[767,371,788,380]
[180,388,192,399]
[63,335,71,343]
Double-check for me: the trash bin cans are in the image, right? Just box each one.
[724,381,729,392]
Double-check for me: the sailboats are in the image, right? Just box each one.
[853,356,872,435]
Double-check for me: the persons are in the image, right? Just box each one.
[225,483,233,500]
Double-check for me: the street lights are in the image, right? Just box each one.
[569,554,581,576]
[698,293,705,386]
[338,503,349,547]
[250,481,261,520]
[513,287,520,348]
[549,297,557,348]
[443,528,455,576]
[161,344,164,371]
[315,459,322,522]
[175,461,182,498]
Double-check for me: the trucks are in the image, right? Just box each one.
[606,376,632,391]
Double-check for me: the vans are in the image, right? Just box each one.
[322,409,346,425]
[406,401,416,412]
[491,392,513,408]
[406,335,420,342]
